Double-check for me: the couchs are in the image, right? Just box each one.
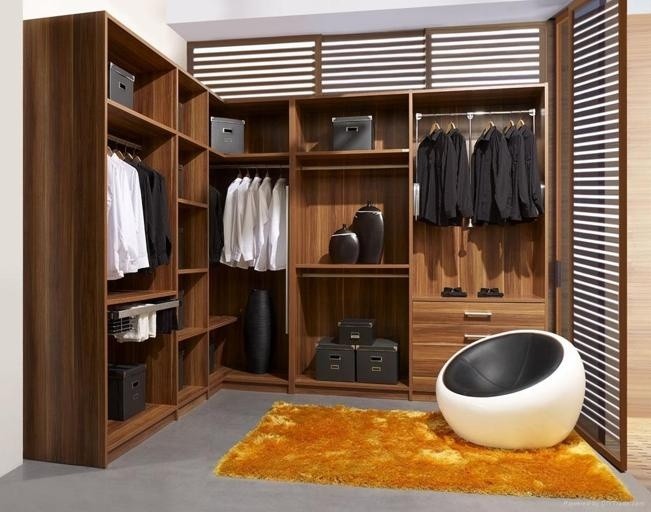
[433,329,586,450]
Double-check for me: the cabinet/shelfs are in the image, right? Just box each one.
[21,11,238,469]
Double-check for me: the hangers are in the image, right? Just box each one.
[104,135,164,182]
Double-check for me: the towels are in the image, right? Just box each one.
[113,304,156,344]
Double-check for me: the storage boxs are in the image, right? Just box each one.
[107,61,136,109]
[210,116,244,155]
[106,360,148,421]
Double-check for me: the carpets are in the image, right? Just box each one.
[212,400,634,501]
